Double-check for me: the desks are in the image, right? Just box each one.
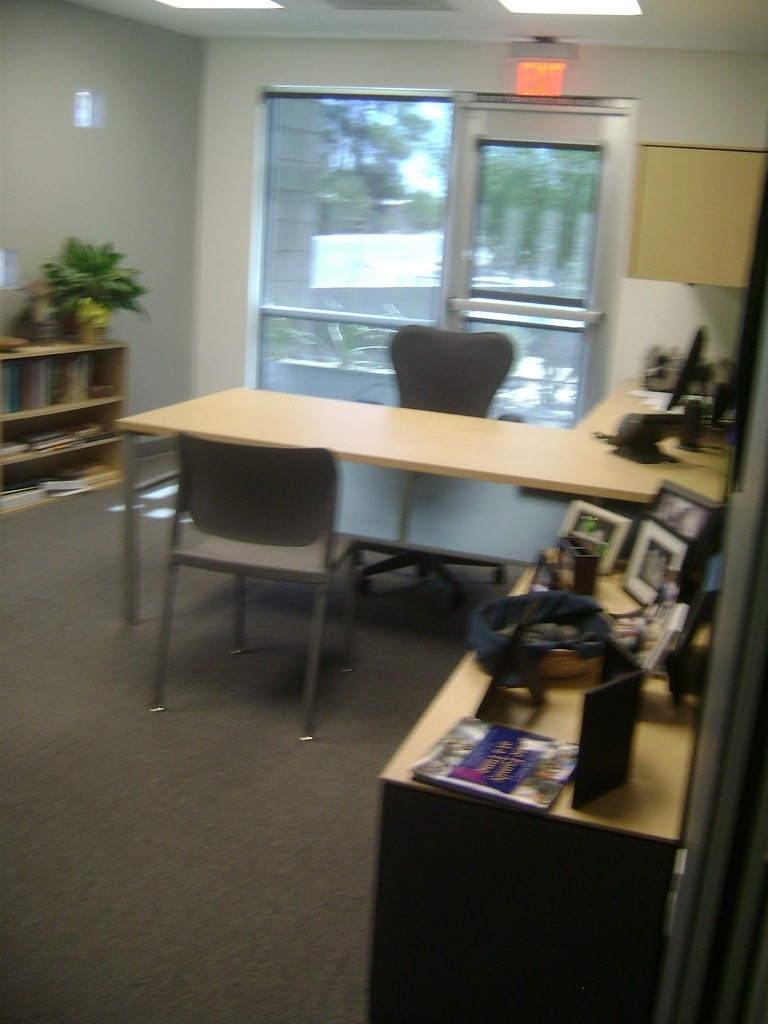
[108,369,768,629]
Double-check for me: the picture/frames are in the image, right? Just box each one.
[478,472,716,820]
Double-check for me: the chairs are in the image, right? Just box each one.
[349,322,517,602]
[143,420,366,745]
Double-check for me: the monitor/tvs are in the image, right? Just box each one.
[666,324,729,431]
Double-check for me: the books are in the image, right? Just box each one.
[410,715,580,812]
[0,354,121,508]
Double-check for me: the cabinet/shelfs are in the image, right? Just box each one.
[620,140,768,293]
[0,336,132,519]
[365,538,738,1024]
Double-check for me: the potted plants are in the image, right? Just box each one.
[37,231,153,349]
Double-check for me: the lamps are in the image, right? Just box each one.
[504,39,584,63]
